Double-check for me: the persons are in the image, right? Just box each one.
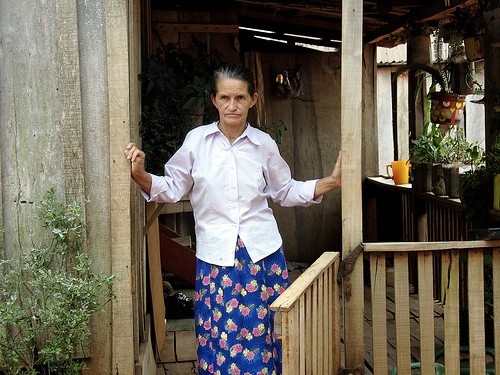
[124,64,342,375]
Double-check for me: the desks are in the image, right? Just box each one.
[142,195,194,361]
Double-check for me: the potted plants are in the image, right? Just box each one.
[385,0,500,222]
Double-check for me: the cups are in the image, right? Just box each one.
[385,160,410,185]
